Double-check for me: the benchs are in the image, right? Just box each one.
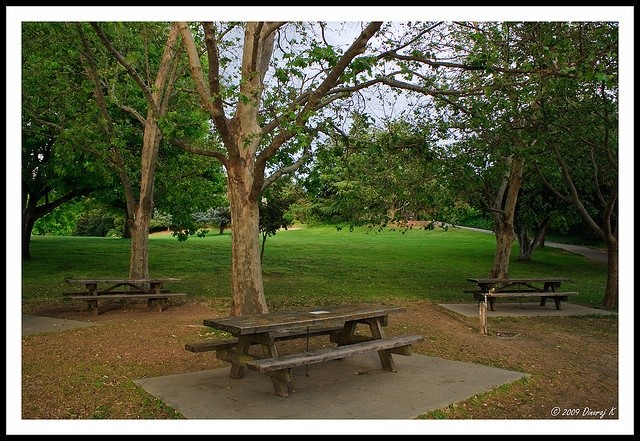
[71,292,186,316]
[63,292,171,302]
[246,334,424,396]
[184,321,355,379]
[471,291,580,312]
[462,289,545,304]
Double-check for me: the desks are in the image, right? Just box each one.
[68,277,178,313]
[467,277,570,309]
[203,303,407,396]
